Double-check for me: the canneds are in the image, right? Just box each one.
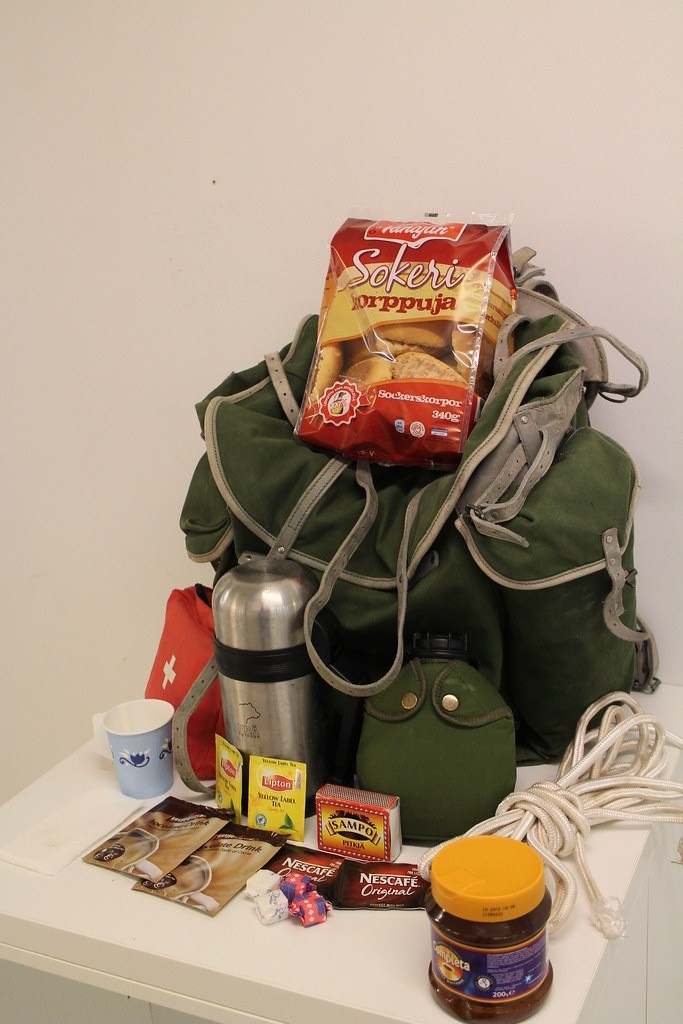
[423,835,554,1024]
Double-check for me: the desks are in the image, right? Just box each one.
[0,682,683,1024]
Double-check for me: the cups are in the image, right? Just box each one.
[102,699,175,799]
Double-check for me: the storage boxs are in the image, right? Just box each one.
[315,784,398,863]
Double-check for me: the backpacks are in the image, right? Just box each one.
[180,247,660,759]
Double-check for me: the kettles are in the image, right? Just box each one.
[212,560,325,818]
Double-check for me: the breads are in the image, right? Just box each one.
[309,318,495,399]
